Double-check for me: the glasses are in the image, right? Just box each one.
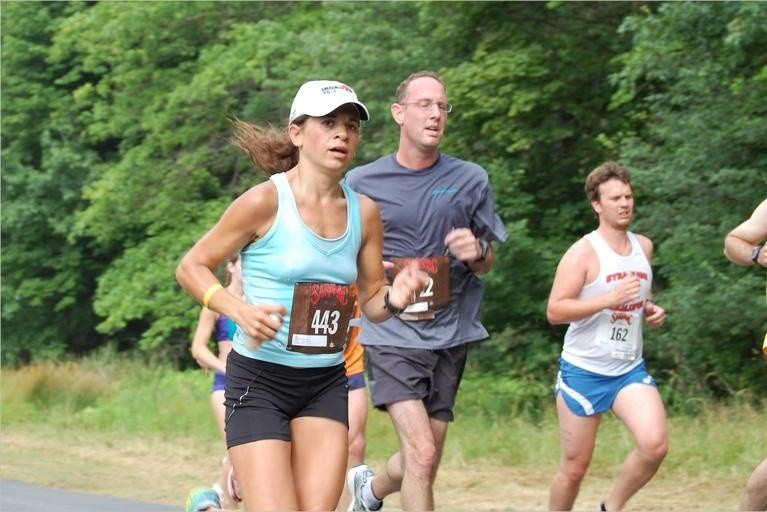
[398,100,453,114]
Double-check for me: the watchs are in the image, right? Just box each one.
[751,245,762,268]
[473,237,490,266]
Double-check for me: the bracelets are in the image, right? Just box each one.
[382,289,406,315]
[205,283,224,308]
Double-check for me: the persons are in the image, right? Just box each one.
[191,253,244,505]
[546,159,669,512]
[343,297,368,477]
[723,195,766,512]
[341,71,510,512]
[184,79,429,511]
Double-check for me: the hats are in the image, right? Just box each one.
[289,80,370,125]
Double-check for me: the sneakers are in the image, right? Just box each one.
[182,487,222,511]
[347,463,383,511]
[212,455,242,511]
